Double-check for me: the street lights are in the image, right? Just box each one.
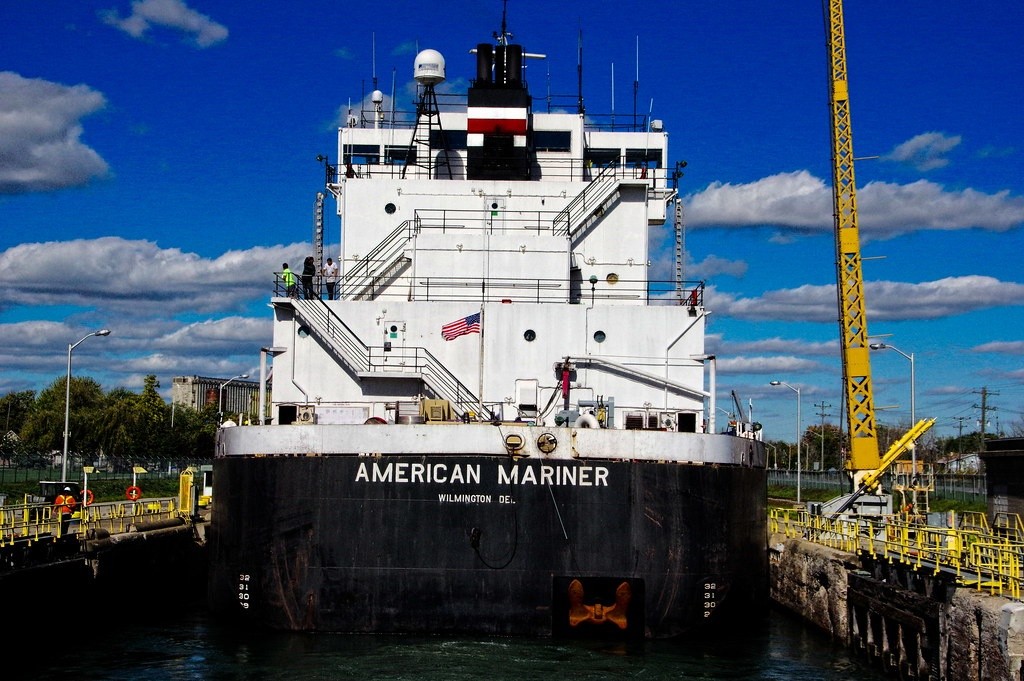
[871,341,921,524]
[219,373,250,427]
[770,380,802,503]
[61,327,111,482]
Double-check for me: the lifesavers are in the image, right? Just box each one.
[127,485,140,501]
[81,489,94,505]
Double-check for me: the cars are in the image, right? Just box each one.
[24,480,83,522]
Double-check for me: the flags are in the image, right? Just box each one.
[441,314,481,341]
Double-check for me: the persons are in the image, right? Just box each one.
[301,256,316,301]
[320,258,338,300]
[53,487,75,534]
[904,503,913,512]
[281,263,295,298]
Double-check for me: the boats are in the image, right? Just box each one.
[214,0,772,661]
